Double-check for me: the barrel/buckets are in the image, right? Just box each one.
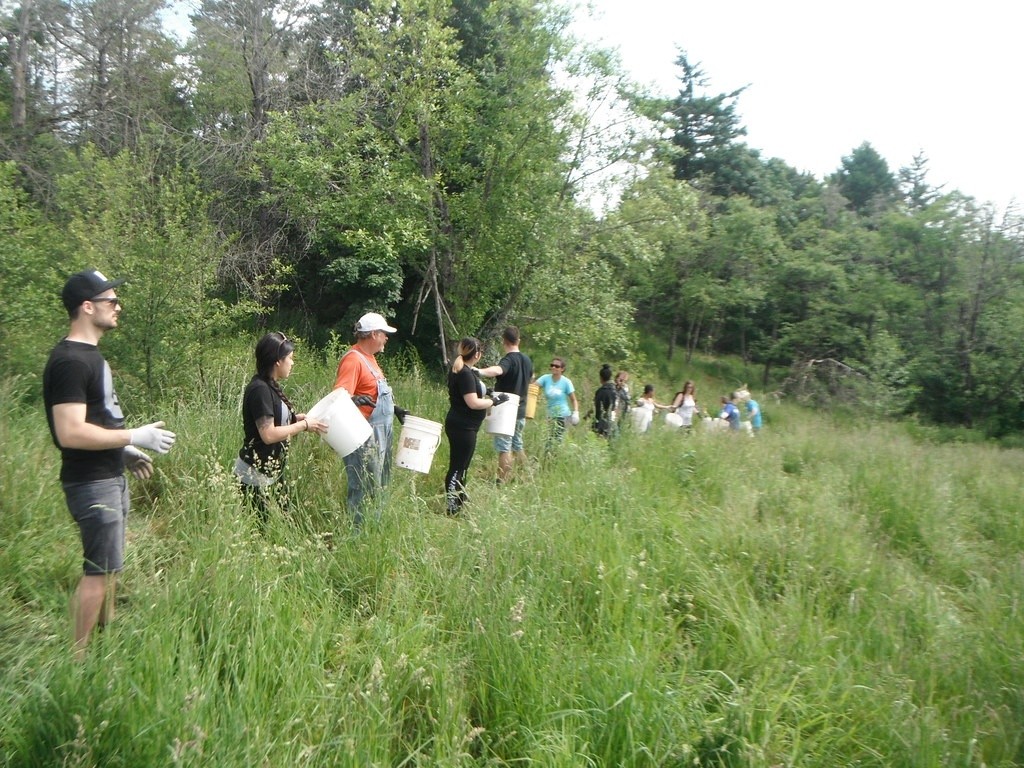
[485,392,521,436]
[396,414,444,474]
[741,420,754,438]
[663,413,683,431]
[303,387,374,458]
[525,384,540,419]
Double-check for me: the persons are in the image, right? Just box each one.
[740,390,762,433]
[533,358,579,457]
[471,325,533,489]
[444,338,510,519]
[592,364,631,440]
[332,312,410,537]
[673,381,699,435]
[234,331,328,535]
[42,269,177,664]
[719,390,740,429]
[639,384,677,429]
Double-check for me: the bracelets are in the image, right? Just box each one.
[304,419,308,431]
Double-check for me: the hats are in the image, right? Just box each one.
[62,269,125,312]
[356,312,397,333]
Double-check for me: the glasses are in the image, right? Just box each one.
[550,364,561,368]
[85,298,121,307]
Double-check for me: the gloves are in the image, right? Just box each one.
[121,445,154,479]
[485,388,492,395]
[697,412,702,419]
[131,421,175,453]
[571,411,580,426]
[351,395,377,409]
[393,405,410,425]
[492,393,509,406]
